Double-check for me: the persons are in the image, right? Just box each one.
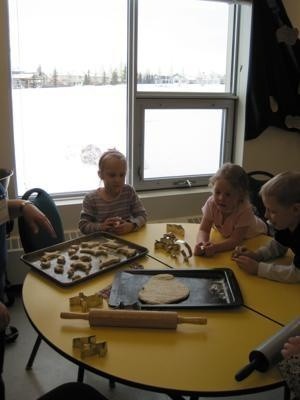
[1,199,58,343]
[78,148,148,235]
[233,170,300,282]
[194,163,269,256]
[278,336,300,400]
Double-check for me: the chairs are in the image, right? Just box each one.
[247,171,274,211]
[18,188,115,389]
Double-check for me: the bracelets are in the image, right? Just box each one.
[19,201,33,207]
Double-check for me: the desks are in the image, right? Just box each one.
[22,223,300,400]
